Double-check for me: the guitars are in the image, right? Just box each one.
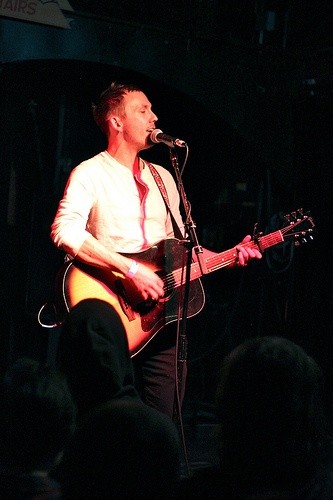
[61,205,316,358]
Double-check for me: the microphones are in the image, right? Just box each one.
[150,129,187,147]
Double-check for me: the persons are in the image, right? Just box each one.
[51,78,262,415]
[161,337,333,500]
[0,357,75,500]
[47,297,182,499]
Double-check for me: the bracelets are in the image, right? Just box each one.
[122,257,139,280]
